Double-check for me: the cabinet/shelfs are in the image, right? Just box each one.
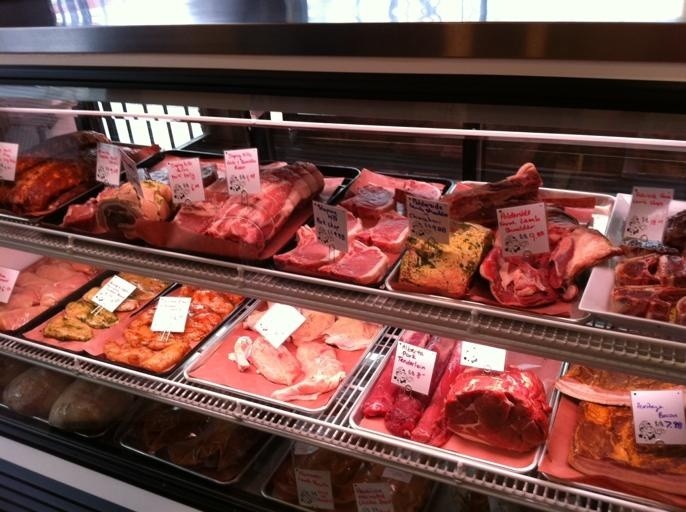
[0,0,686,512]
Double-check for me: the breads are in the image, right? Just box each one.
[272,441,432,510]
[127,402,266,483]
[1,353,32,390]
[2,365,76,415]
[48,379,134,430]
